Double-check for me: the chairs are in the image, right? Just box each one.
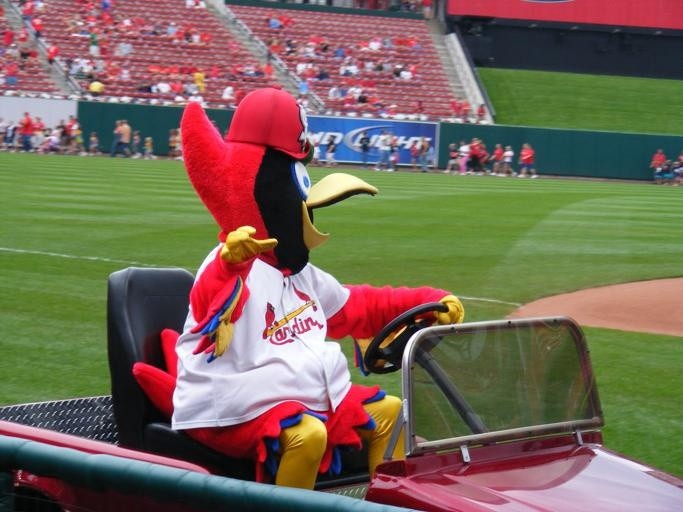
[107,266,352,481]
[0,0,459,117]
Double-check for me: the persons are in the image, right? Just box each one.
[670,153,682,186]
[648,147,667,184]
[659,159,674,186]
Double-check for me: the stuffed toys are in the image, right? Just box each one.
[129,84,467,496]
